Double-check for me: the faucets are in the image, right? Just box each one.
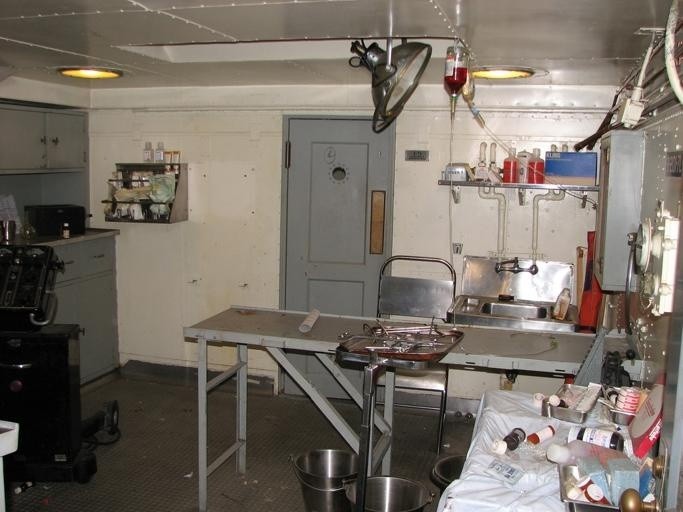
[495,256,538,275]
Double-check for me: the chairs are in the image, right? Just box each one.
[363,255,457,456]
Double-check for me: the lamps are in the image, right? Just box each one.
[348,1,432,133]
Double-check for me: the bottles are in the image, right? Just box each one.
[574,482,604,504]
[566,424,627,451]
[526,424,557,444]
[502,427,526,452]
[552,288,571,321]
[504,148,544,184]
[142,141,166,164]
[545,438,631,468]
[113,164,179,190]
[62,222,71,240]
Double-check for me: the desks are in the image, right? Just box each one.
[184,308,597,511]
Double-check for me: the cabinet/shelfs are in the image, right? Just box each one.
[0,104,88,174]
[41,235,120,397]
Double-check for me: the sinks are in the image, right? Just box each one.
[479,300,549,320]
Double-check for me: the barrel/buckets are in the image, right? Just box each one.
[341,476,434,512]
[285,448,361,512]
[431,453,468,487]
